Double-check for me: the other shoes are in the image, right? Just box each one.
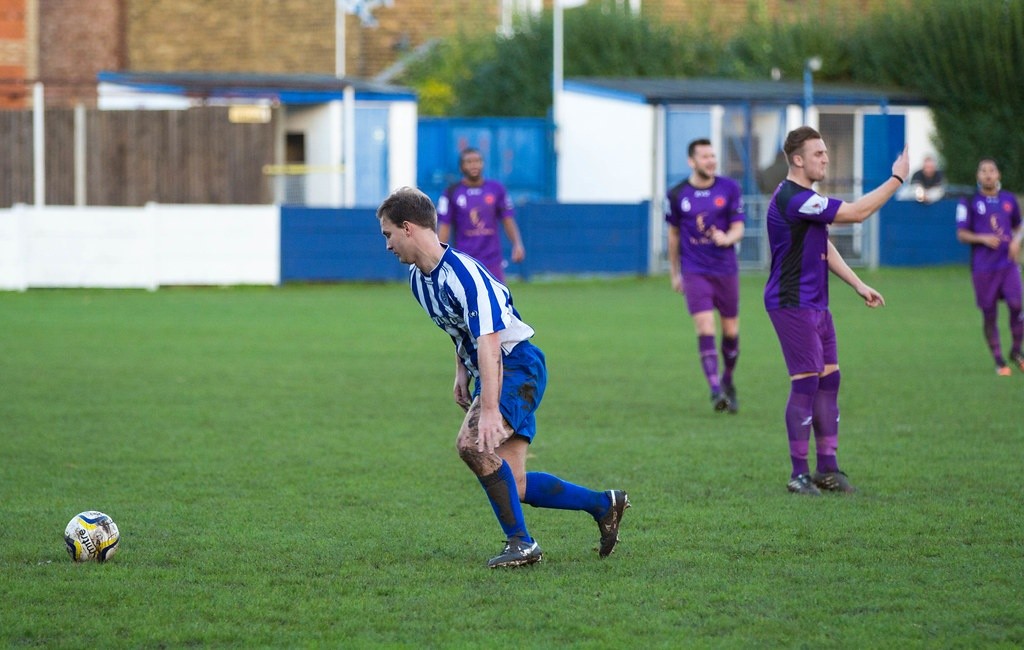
[725,387,737,413]
[710,389,726,411]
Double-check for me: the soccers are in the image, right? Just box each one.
[64,509,121,563]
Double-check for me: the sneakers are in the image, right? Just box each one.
[812,469,855,492]
[595,490,632,559]
[1009,351,1024,371]
[787,472,823,496]
[488,535,542,567]
[995,365,1011,376]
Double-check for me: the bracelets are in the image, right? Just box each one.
[891,175,903,183]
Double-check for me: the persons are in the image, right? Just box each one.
[436,146,524,285]
[376,187,629,568]
[955,157,1024,377]
[764,126,911,495]
[662,139,747,415]
[912,156,948,205]
